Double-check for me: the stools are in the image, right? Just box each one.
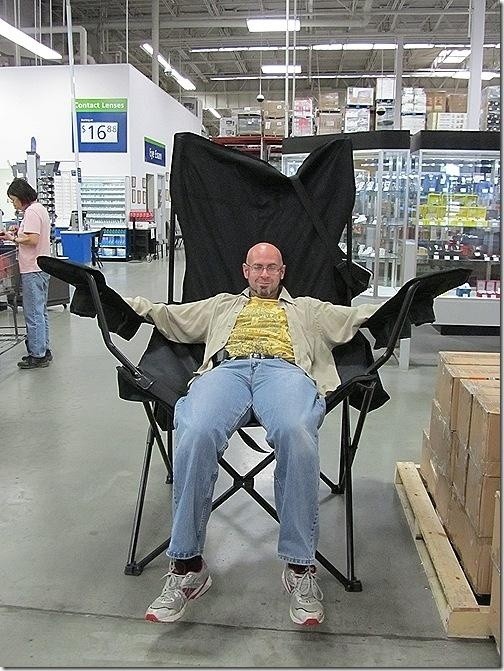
[149,238,168,260]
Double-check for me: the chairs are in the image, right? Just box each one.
[92,227,107,269]
[38,132,472,593]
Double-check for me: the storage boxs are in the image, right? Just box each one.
[420,351,500,651]
[219,79,500,137]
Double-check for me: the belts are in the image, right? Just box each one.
[225,353,296,367]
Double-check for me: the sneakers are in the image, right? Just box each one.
[21,350,52,362]
[144,556,212,624]
[281,565,326,626]
[16,355,49,369]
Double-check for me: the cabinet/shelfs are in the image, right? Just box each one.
[281,129,500,371]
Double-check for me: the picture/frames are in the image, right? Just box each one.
[132,177,147,204]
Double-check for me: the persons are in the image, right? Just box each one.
[121,243,390,625]
[0,176,54,371]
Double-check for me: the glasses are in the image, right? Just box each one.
[244,262,283,276]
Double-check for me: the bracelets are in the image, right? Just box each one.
[13,238,15,242]
